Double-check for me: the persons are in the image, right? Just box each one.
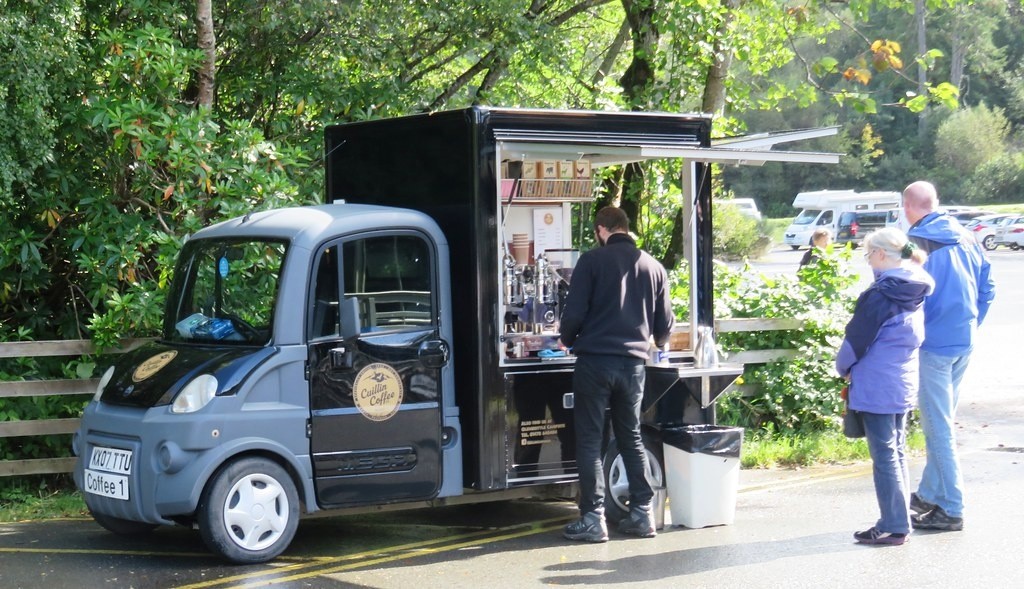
[903,180,997,534]
[796,229,829,286]
[835,227,936,547]
[559,205,675,543]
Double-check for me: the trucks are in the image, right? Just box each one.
[71,100,737,568]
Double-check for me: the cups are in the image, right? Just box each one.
[512,233,529,265]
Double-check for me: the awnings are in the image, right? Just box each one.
[714,124,847,166]
[498,138,847,226]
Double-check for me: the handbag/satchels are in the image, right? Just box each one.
[842,387,867,438]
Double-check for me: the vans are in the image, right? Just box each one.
[778,188,981,248]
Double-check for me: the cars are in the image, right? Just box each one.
[994,215,1024,250]
[965,214,1020,251]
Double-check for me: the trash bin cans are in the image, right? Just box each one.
[662,424,744,527]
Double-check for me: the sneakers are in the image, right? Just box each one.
[562,506,609,542]
[618,501,658,538]
[910,505,964,531]
[855,525,907,546]
[909,493,935,514]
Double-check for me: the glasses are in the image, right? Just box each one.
[864,249,881,261]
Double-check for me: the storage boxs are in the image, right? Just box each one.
[521,161,593,197]
[176,313,236,341]
